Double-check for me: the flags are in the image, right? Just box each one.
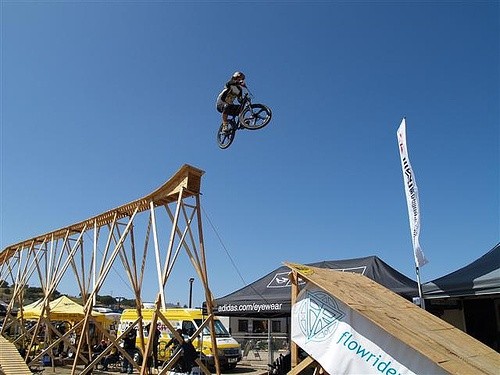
[396,116,429,269]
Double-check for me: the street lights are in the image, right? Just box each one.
[189,276,196,307]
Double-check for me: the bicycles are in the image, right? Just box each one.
[216,92,272,149]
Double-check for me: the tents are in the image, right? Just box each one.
[1,295,104,365]
[420,242,500,354]
[198,256,422,375]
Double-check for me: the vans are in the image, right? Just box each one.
[117,307,243,371]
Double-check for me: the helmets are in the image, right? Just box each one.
[233,72,245,80]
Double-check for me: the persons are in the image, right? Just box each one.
[92,332,119,370]
[164,329,199,375]
[215,72,250,133]
[146,324,162,369]
[120,322,137,375]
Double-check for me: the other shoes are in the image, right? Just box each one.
[222,122,229,131]
[121,369,133,374]
[101,367,108,371]
[240,120,250,127]
